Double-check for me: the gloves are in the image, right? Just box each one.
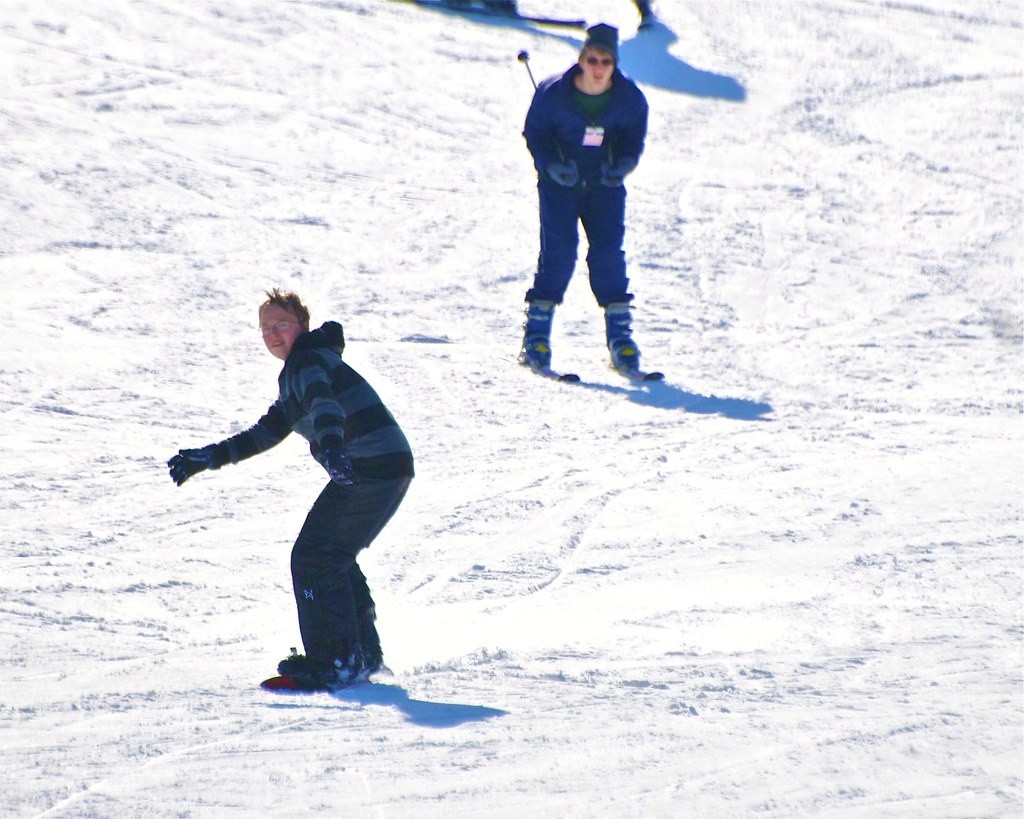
[167,441,223,487]
[545,159,580,188]
[601,154,636,187]
[320,448,362,491]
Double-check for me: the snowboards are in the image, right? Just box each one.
[261,657,372,691]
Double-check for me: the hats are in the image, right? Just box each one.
[584,23,620,51]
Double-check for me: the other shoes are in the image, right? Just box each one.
[364,643,387,674]
[277,641,363,685]
[638,10,657,29]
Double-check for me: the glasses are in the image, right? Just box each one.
[584,55,614,67]
[258,320,299,336]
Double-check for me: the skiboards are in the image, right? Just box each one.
[530,362,666,382]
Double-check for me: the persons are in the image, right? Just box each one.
[167,288,414,678]
[519,22,650,373]
[633,0,656,28]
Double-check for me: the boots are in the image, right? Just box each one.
[523,304,554,366]
[603,302,640,365]
